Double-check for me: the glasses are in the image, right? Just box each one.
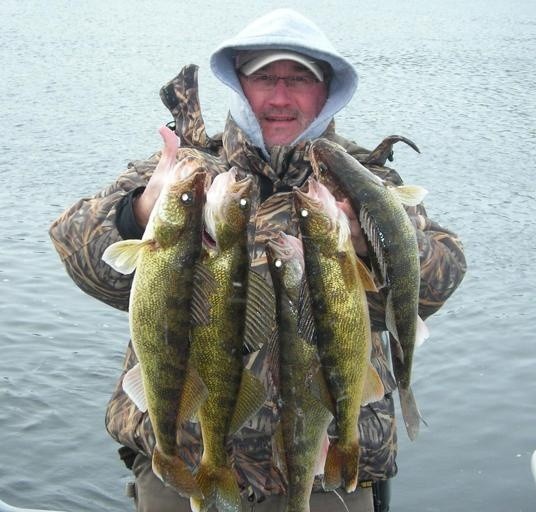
[242,72,321,90]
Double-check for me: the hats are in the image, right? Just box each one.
[234,48,325,82]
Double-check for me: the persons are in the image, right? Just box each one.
[47,9,470,512]
